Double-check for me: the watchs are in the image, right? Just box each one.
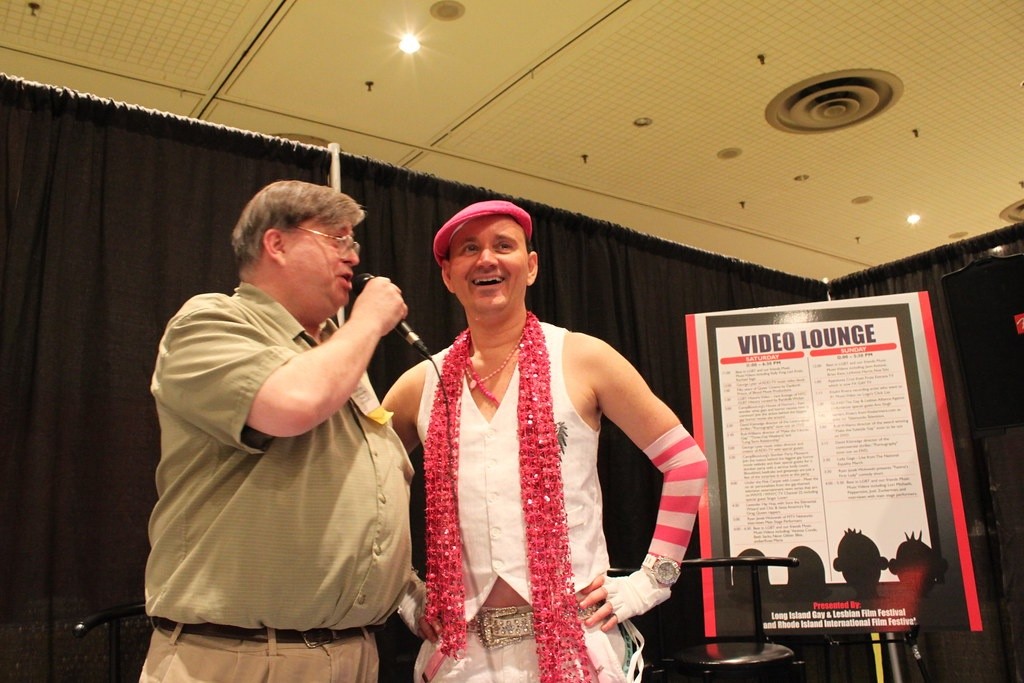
[643,557,681,585]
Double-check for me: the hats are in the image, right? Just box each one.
[432,200,533,266]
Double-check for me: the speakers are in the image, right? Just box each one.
[940,252,1024,439]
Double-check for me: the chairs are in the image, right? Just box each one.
[659,557,799,683]
[73,603,145,683]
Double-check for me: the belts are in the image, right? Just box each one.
[150,615,377,650]
[466,603,608,652]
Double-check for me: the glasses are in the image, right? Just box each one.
[295,224,362,260]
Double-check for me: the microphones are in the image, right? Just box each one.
[355,274,432,360]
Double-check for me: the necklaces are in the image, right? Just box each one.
[466,334,524,406]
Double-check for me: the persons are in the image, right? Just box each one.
[381,201,708,683]
[138,180,415,683]
